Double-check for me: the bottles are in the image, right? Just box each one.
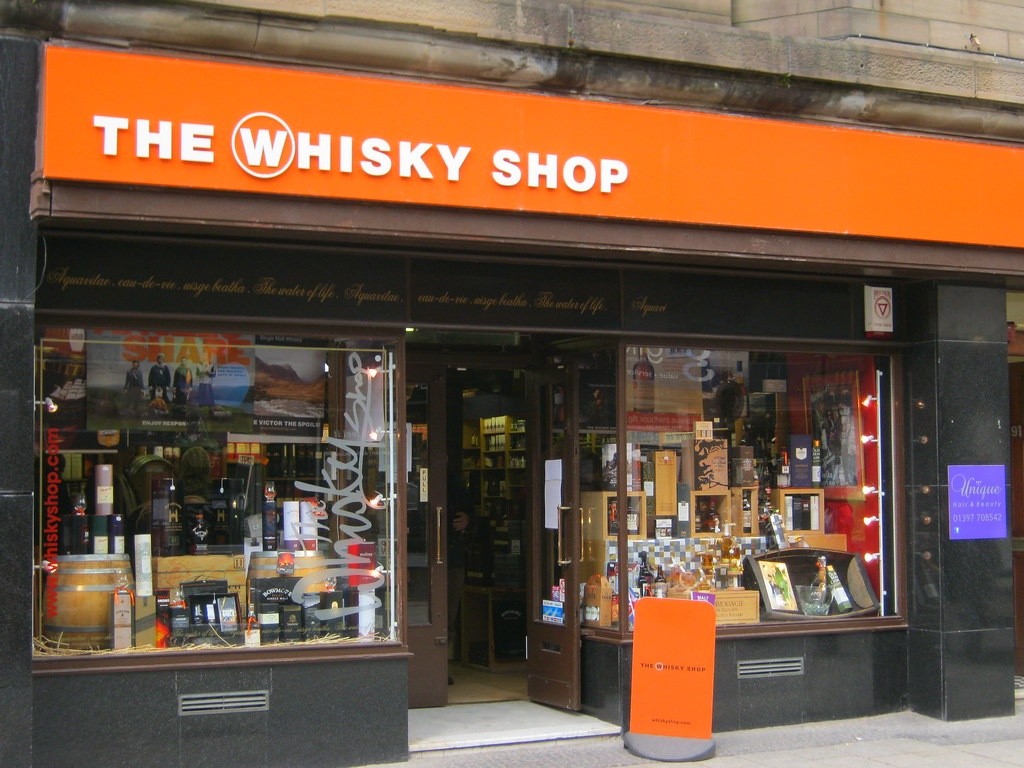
[695,497,721,532]
[654,565,667,598]
[812,440,821,488]
[769,441,790,487]
[722,525,733,564]
[729,538,740,567]
[607,555,616,585]
[743,490,752,533]
[702,543,713,574]
[639,551,652,597]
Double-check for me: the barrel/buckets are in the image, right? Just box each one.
[44,555,135,649]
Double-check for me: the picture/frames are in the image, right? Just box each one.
[748,557,806,619]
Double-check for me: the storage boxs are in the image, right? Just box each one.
[731,446,754,487]
[682,439,728,491]
[653,449,678,517]
[790,435,812,489]
[185,589,243,635]
[247,578,360,643]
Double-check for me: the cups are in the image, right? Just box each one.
[795,586,835,616]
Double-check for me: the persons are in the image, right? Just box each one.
[172,357,193,407]
[195,353,217,417]
[147,355,172,402]
[715,373,744,409]
[422,450,476,685]
[121,359,145,415]
[812,397,858,484]
